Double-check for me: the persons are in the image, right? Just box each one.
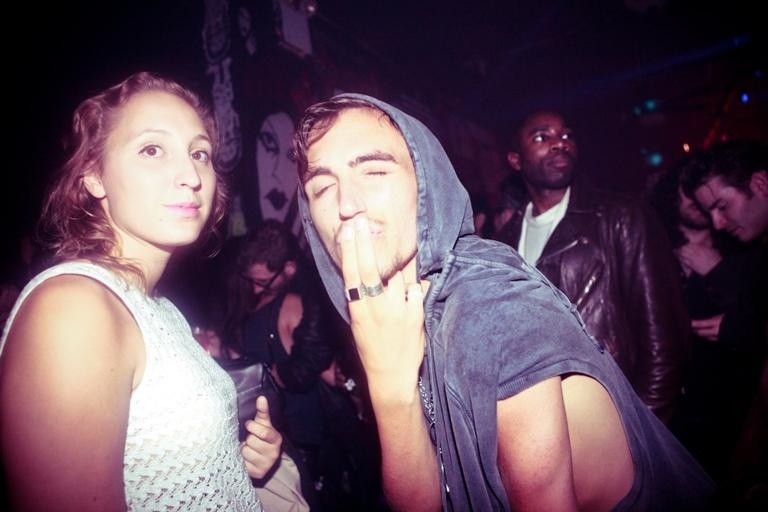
[291,93,706,512]
[481,112,765,512]
[224,221,375,511]
[1,69,282,512]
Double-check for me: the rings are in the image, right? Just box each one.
[367,283,384,297]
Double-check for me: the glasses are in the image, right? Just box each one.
[234,249,299,292]
